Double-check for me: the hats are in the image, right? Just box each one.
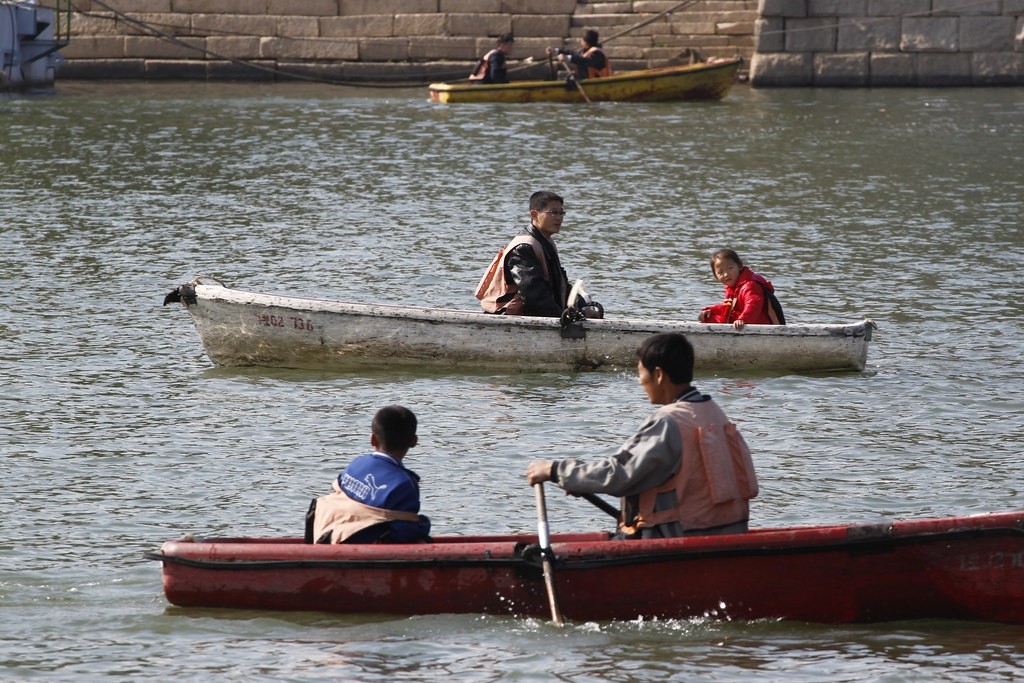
[582,30,599,44]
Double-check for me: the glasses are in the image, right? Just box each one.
[538,209,565,216]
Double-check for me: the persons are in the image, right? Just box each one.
[299,406,431,545]
[698,251,785,332]
[470,34,515,85]
[545,31,610,79]
[526,334,758,540]
[473,190,605,318]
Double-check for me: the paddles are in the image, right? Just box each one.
[532,480,568,629]
[555,47,593,104]
[560,473,624,523]
[548,49,556,81]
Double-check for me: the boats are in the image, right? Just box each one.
[141,506,1024,629]
[427,46,743,104]
[161,274,878,378]
